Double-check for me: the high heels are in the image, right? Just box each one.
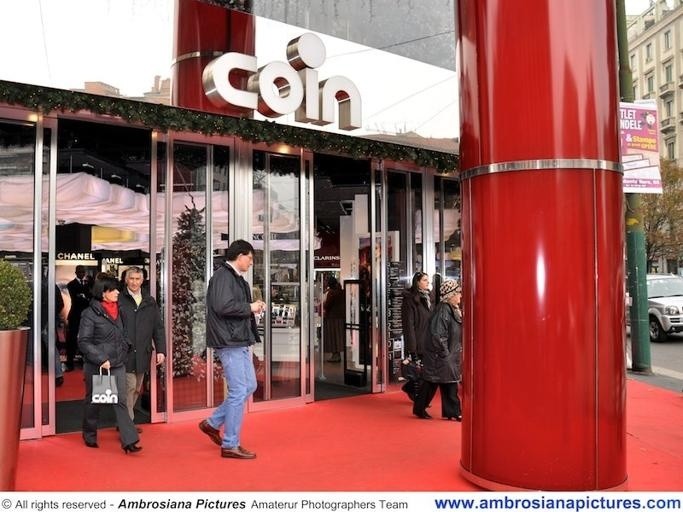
[413,411,431,419]
[83,436,98,447]
[124,444,142,454]
[448,414,462,421]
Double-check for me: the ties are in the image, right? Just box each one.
[80,280,83,285]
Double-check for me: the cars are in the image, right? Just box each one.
[625,273,683,343]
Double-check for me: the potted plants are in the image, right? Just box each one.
[0,256,33,492]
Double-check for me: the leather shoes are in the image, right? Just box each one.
[116,426,142,433]
[221,446,256,459]
[402,386,414,401]
[199,419,222,447]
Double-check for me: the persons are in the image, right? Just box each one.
[323,277,345,362]
[22,264,198,453]
[401,271,463,422]
[200,240,267,458]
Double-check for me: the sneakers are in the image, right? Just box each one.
[327,353,341,362]
[55,357,84,386]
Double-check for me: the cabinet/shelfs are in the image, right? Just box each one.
[254,263,341,364]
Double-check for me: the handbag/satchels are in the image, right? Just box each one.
[402,360,424,381]
[90,375,119,405]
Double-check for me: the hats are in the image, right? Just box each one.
[439,280,459,300]
